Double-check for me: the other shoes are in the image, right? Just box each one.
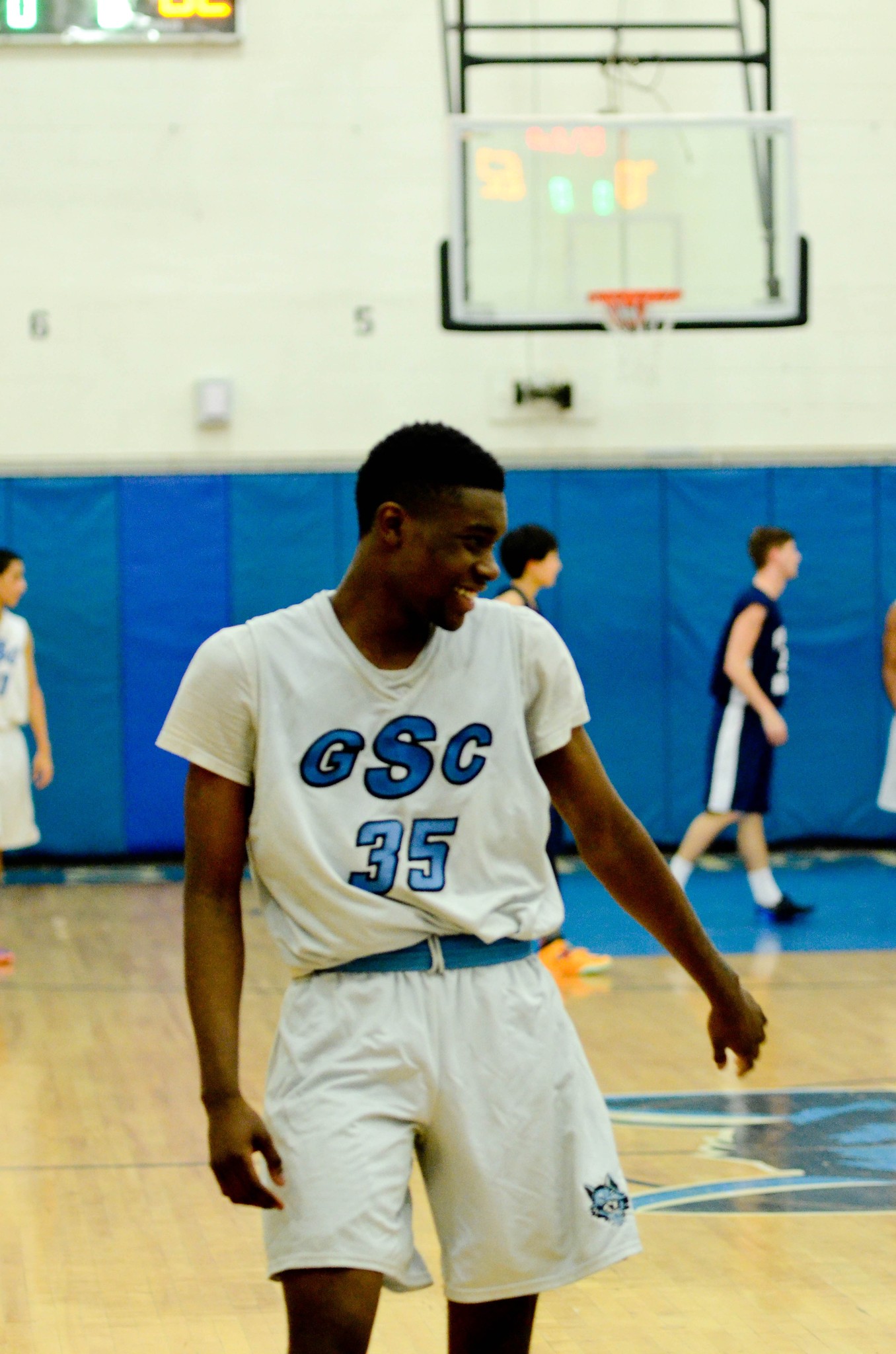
[756,895,814,928]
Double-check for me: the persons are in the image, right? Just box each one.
[880,600,896,826]
[0,547,55,966]
[670,526,816,930]
[156,421,767,1354]
[493,525,616,978]
